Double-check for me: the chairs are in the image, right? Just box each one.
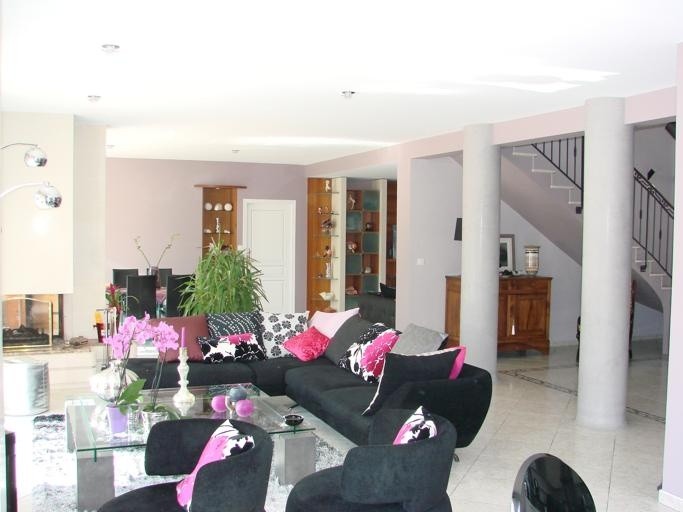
[508,449,594,511]
[93,416,276,512]
[284,406,461,511]
[105,267,196,315]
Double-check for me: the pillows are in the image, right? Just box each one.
[359,347,461,419]
[392,406,436,447]
[284,309,467,383]
[171,418,253,509]
[144,310,310,362]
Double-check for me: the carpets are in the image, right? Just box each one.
[29,409,346,512]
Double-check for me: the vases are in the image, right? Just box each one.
[88,362,142,401]
[141,410,167,442]
[107,405,129,435]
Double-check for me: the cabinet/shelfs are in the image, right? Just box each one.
[193,185,247,266]
[307,177,339,311]
[347,190,379,296]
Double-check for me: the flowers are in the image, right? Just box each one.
[105,283,140,361]
[100,312,161,414]
[142,320,182,419]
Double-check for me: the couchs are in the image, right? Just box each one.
[112,308,494,467]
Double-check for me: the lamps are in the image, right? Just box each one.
[0,180,63,208]
[1,140,46,170]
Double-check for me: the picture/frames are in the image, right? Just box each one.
[498,233,515,273]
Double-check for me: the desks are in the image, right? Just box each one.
[444,273,553,354]
